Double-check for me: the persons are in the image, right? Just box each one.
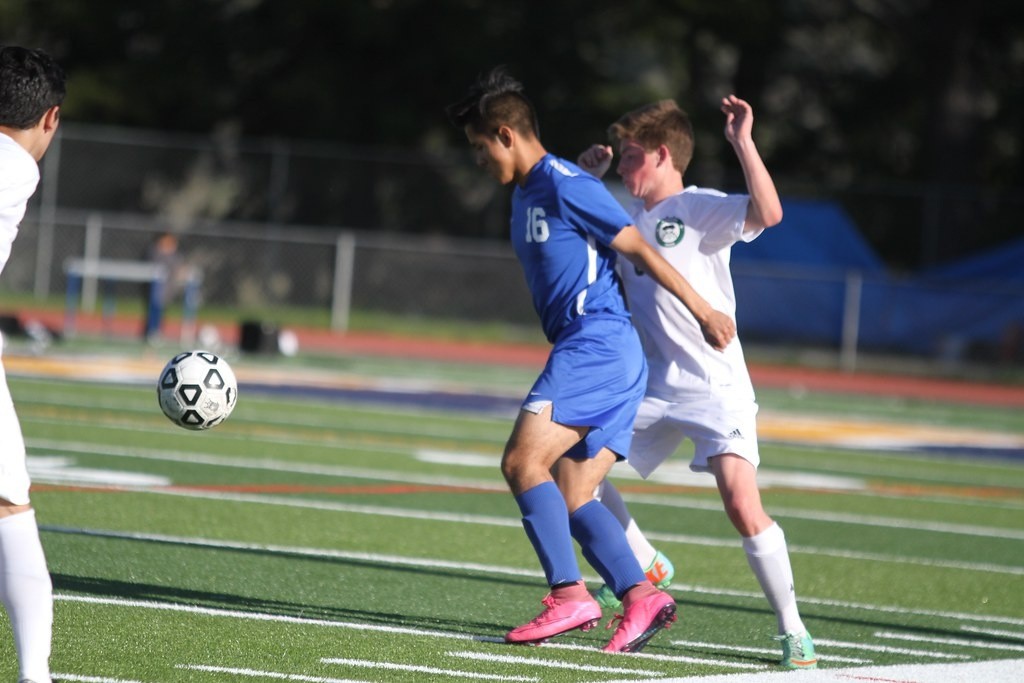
[0,45,67,683]
[141,233,186,347]
[453,69,736,653]
[576,95,816,671]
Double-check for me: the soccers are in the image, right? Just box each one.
[156,350,239,432]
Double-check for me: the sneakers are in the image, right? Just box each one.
[592,550,674,609]
[505,583,602,646]
[604,585,676,653]
[771,630,818,669]
